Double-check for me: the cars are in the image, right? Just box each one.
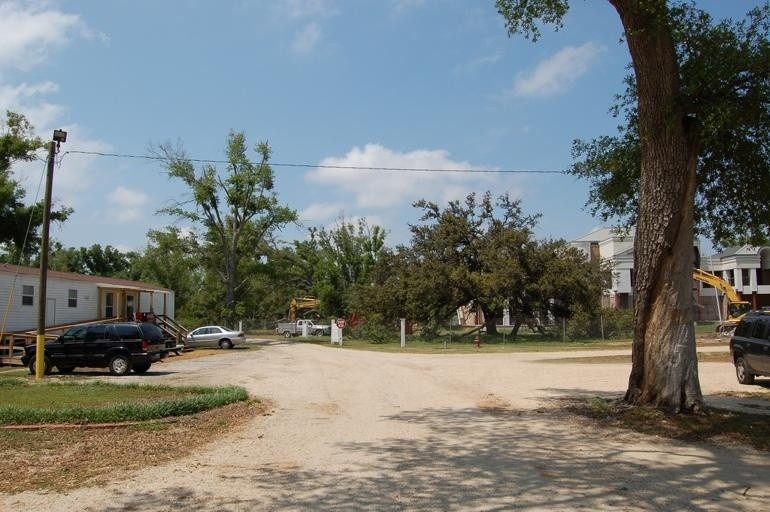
[184,326,248,350]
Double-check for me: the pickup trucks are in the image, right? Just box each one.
[277,321,331,339]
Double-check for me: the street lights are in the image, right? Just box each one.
[35,124,68,377]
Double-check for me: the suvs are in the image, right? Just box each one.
[730,313,770,385]
[23,322,172,378]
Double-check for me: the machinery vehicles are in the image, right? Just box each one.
[692,265,769,334]
[287,297,322,324]
